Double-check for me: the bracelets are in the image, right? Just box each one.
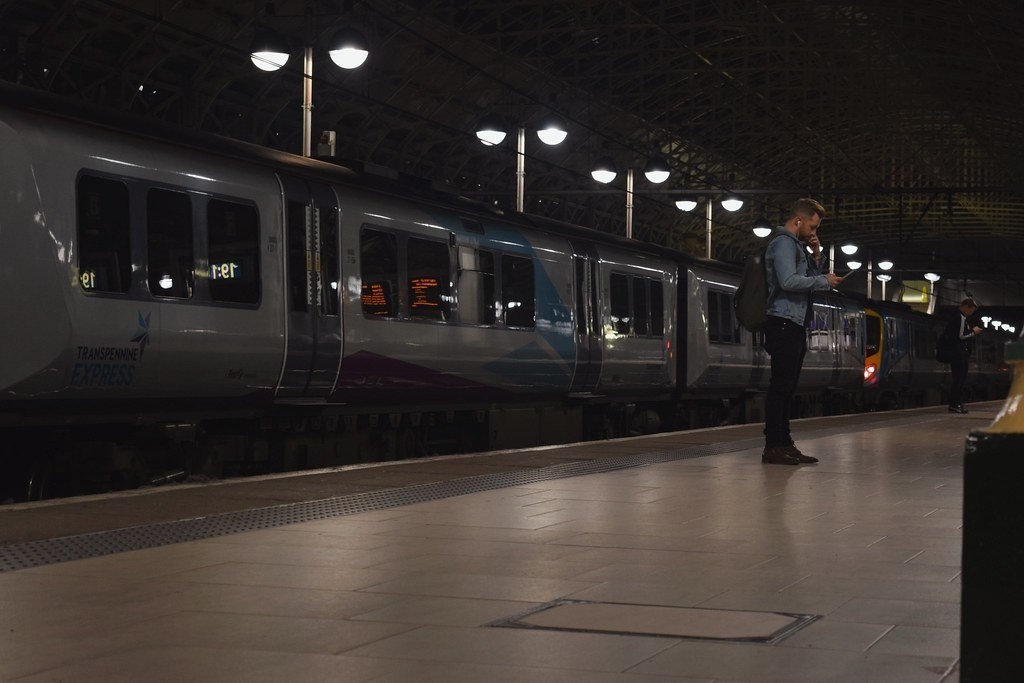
[813,250,822,261]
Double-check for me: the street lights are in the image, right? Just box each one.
[478,109,570,215]
[590,153,673,240]
[675,183,744,260]
[809,237,860,278]
[248,25,369,160]
[846,248,894,299]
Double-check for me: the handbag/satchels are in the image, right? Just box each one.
[935,334,964,363]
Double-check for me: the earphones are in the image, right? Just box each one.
[797,221,801,224]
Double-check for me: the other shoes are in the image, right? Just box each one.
[784,445,818,463]
[948,402,969,413]
[761,445,800,465]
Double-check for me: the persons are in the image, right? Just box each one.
[948,298,983,413]
[761,197,844,465]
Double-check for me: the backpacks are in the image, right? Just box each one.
[734,232,801,333]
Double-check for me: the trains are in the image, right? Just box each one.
[0,98,1023,471]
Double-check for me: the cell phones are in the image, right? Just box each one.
[843,271,854,279]
